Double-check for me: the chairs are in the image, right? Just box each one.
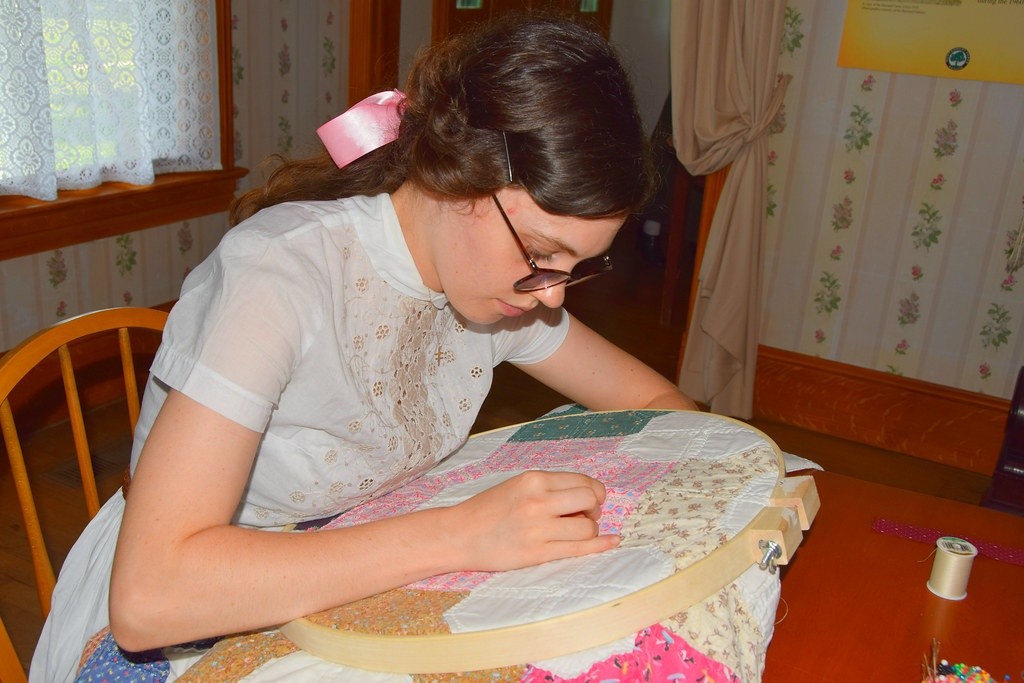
[0,305,172,683]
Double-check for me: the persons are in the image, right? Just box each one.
[25,9,700,683]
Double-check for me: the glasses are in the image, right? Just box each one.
[490,189,614,291]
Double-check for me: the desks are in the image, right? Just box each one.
[747,420,1024,683]
